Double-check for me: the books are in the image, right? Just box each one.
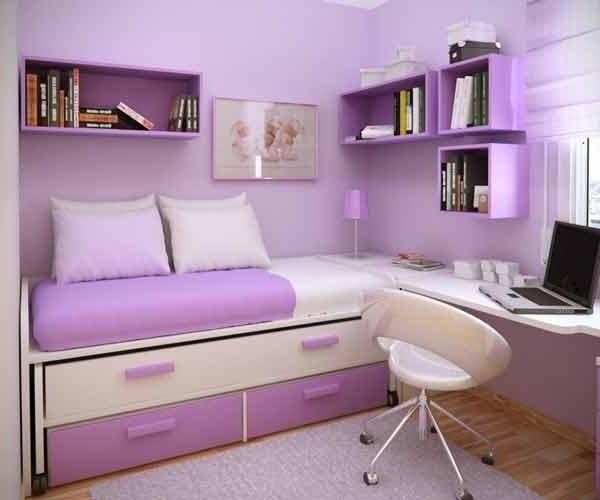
[21,62,198,132]
[359,68,490,211]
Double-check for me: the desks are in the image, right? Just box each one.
[321,254,600,342]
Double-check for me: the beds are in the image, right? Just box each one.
[19,248,389,500]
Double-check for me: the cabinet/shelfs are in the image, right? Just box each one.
[43,318,390,488]
[339,52,529,220]
[20,55,203,139]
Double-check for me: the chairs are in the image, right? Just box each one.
[358,288,513,500]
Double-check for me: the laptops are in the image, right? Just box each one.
[478,218,600,314]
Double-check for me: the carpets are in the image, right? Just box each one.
[87,408,544,500]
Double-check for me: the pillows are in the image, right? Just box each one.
[47,190,273,290]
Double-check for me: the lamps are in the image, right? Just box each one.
[344,190,368,260]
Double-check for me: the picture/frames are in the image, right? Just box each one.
[211,96,320,181]
